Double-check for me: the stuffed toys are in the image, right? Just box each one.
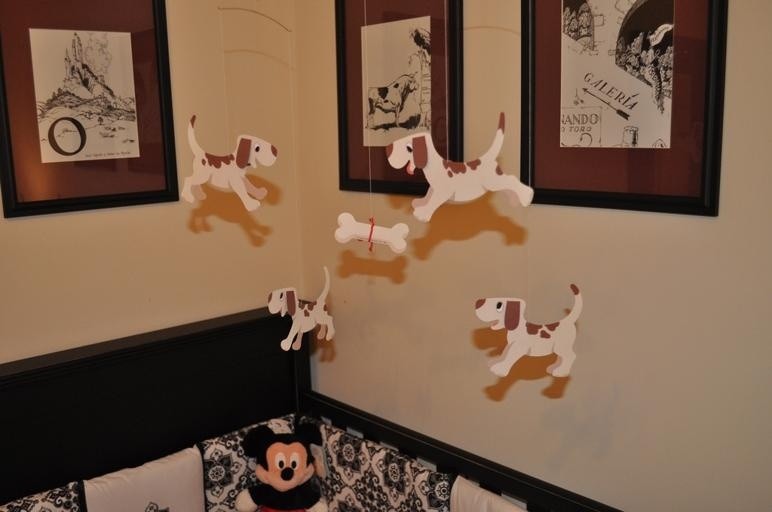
[229,420,331,512]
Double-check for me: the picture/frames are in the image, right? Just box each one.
[520,0,729,216]
[0,0,179,218]
[334,0,464,196]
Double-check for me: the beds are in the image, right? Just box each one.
[1,302,626,512]
